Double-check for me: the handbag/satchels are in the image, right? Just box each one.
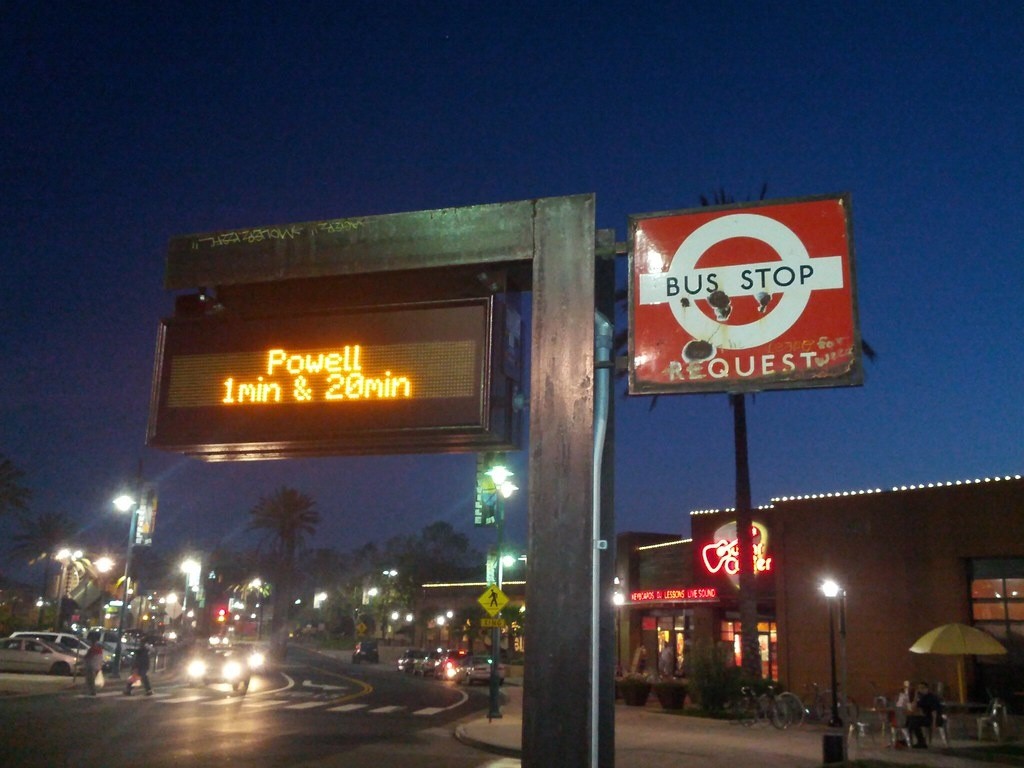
[129,673,142,687]
[94,670,105,688]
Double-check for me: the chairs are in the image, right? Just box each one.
[873,696,896,743]
[845,695,875,748]
[977,698,1001,741]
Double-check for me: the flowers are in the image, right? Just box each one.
[614,670,653,685]
[651,672,689,687]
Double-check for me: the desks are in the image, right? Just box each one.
[939,702,990,742]
[864,706,908,747]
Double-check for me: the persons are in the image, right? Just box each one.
[658,641,673,672]
[81,638,103,695]
[638,644,648,673]
[123,640,153,695]
[896,680,944,749]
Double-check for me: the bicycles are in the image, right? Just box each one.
[801,679,860,725]
[869,679,895,747]
[730,681,806,731]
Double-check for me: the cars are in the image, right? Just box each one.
[433,649,467,680]
[0,626,184,676]
[351,641,379,665]
[454,655,507,685]
[412,649,450,677]
[186,633,268,690]
[396,649,427,673]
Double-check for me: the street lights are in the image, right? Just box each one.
[92,547,113,626]
[612,578,625,677]
[111,488,140,678]
[819,579,842,726]
[485,452,528,719]
[179,555,196,665]
[55,547,83,630]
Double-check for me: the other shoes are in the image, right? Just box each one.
[145,691,153,696]
[913,743,929,750]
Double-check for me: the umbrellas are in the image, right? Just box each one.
[909,623,1007,703]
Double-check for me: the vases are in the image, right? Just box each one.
[620,684,647,707]
[653,687,684,709]
